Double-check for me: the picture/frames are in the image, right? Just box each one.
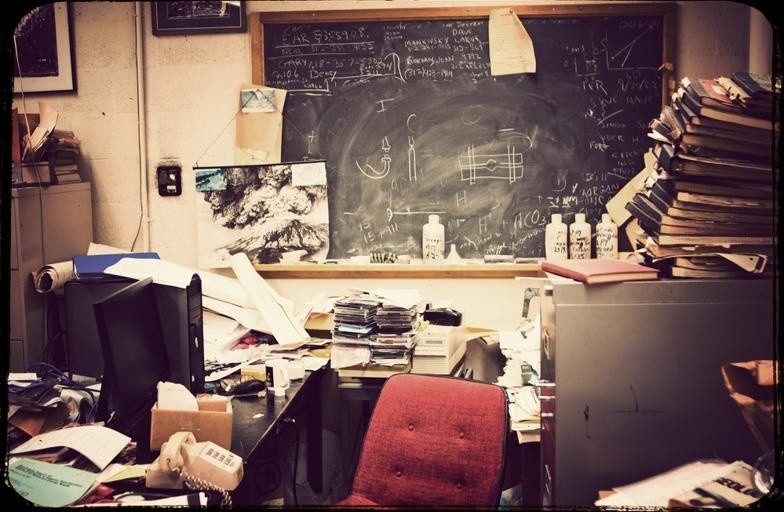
[1,0,76,98]
[150,0,247,37]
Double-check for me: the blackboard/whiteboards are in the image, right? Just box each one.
[247,1,674,280]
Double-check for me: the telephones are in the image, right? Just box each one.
[146,432,244,491]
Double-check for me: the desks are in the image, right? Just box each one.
[6,327,329,507]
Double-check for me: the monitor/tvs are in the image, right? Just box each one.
[94,279,171,433]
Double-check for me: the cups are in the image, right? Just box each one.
[263,356,298,398]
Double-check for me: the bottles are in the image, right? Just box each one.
[422,214,445,264]
[594,213,621,265]
[568,213,593,262]
[544,212,568,263]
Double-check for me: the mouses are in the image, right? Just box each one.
[234,379,265,396]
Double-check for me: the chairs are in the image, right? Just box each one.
[335,371,508,511]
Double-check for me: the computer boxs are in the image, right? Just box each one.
[51,274,206,395]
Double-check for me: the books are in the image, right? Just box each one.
[538,258,661,285]
[327,287,544,446]
[623,70,782,279]
[7,372,137,509]
[593,450,773,512]
[10,102,83,184]
